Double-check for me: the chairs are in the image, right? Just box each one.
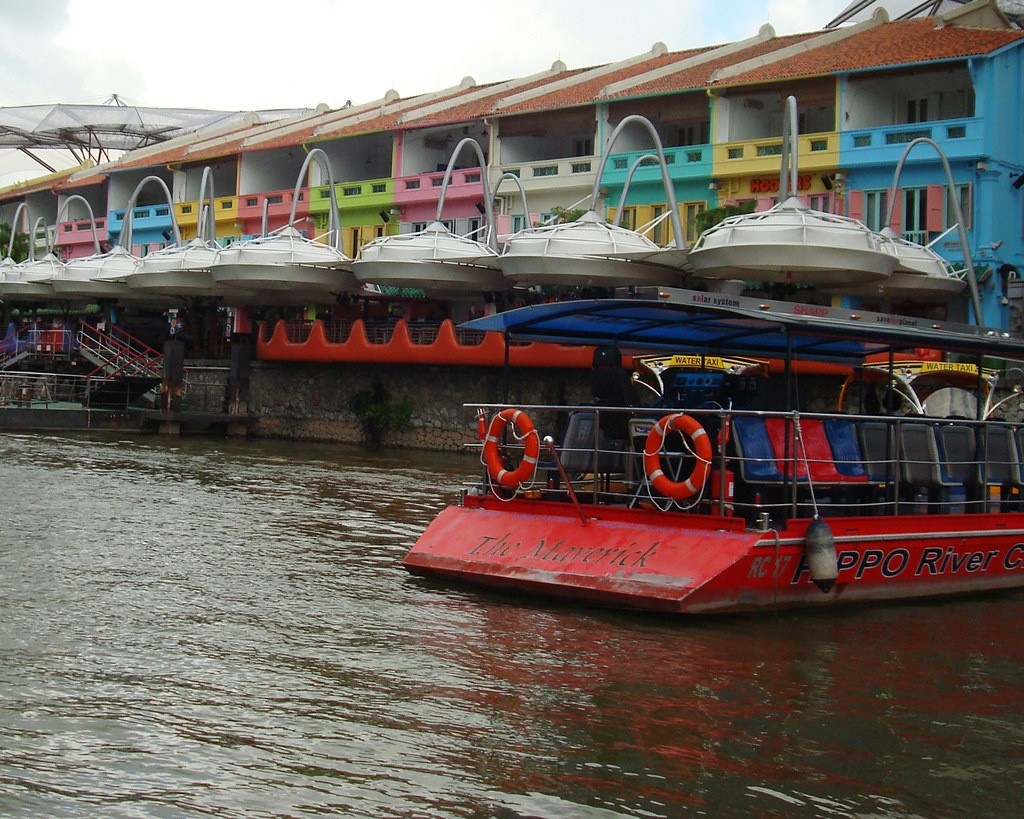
[732,415,1024,520]
[540,410,686,511]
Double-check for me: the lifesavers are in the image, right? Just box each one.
[482,407,541,483]
[645,413,714,499]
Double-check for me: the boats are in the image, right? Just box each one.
[400,285,1024,617]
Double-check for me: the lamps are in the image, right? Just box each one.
[379,209,390,223]
[162,231,170,240]
[821,174,835,190]
[52,250,58,257]
[1010,172,1024,189]
[475,202,485,215]
[100,245,106,253]
